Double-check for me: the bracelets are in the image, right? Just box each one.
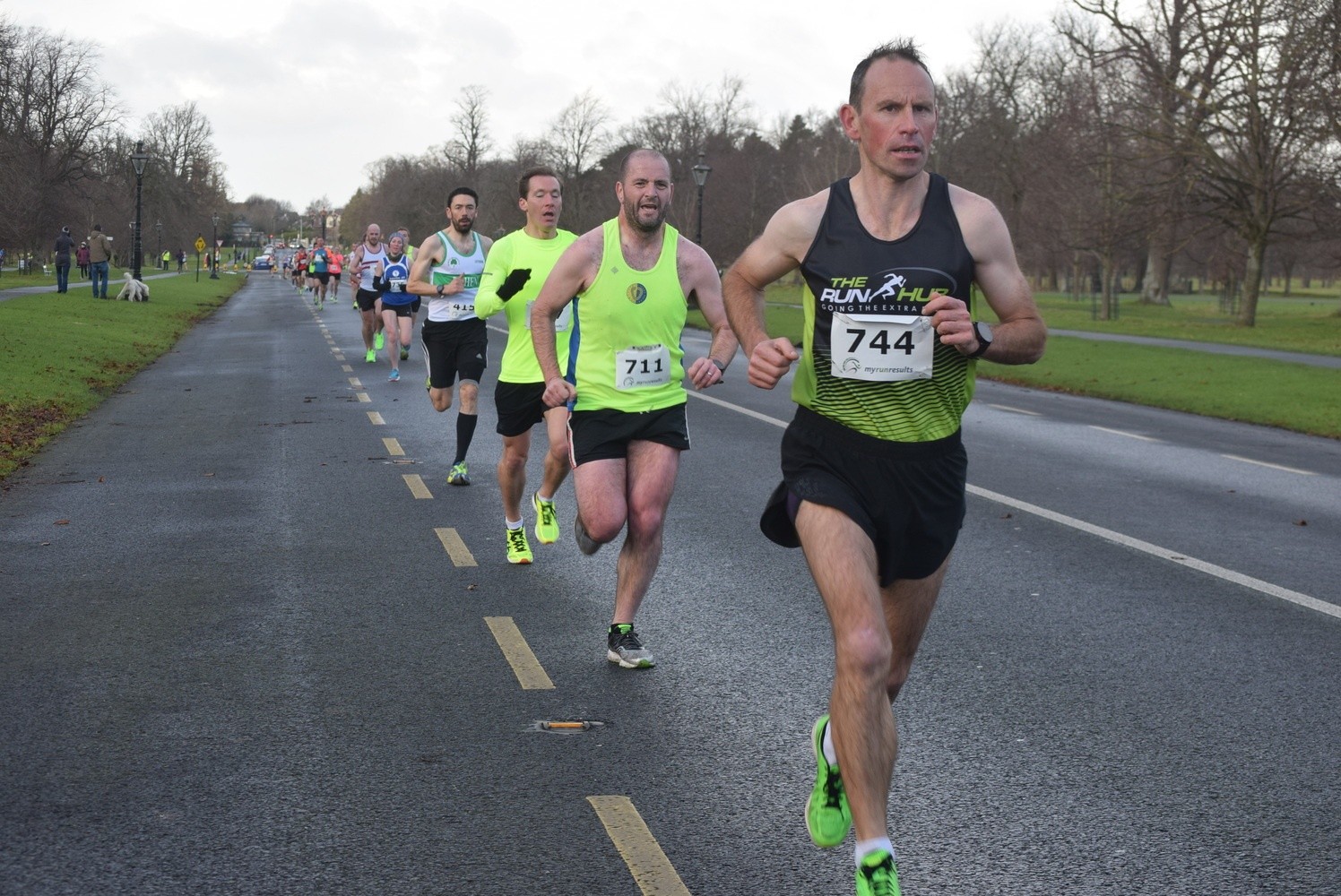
[712,359,725,376]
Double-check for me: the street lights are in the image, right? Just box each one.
[332,214,337,250]
[209,211,219,281]
[125,138,150,301]
[155,219,163,268]
[687,149,713,311]
[216,238,223,272]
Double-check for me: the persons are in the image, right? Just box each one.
[87,245,93,281]
[161,248,171,271]
[396,225,423,330]
[405,184,498,484]
[372,231,417,383]
[530,146,740,675]
[474,167,585,568]
[74,245,82,269]
[77,241,88,279]
[201,222,372,318]
[350,222,392,365]
[89,224,112,299]
[182,250,189,272]
[52,226,76,292]
[176,248,184,272]
[721,35,1057,896]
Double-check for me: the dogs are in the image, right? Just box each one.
[115,272,149,302]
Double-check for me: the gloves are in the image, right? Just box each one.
[373,276,391,290]
[495,269,532,302]
[399,284,406,293]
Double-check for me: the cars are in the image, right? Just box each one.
[254,236,300,269]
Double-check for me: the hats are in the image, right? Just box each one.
[81,242,86,245]
[62,226,69,233]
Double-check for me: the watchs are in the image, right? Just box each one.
[967,319,996,362]
[436,284,445,296]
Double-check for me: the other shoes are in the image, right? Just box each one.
[58,289,62,293]
[94,295,99,298]
[88,277,93,280]
[100,295,107,299]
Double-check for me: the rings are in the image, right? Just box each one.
[706,372,712,376]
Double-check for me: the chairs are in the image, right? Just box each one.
[43,265,52,276]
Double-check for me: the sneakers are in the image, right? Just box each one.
[388,368,400,382]
[334,296,337,302]
[574,513,601,554]
[366,349,376,362]
[376,331,384,349]
[531,491,559,545]
[506,525,533,564]
[400,345,410,360]
[314,296,318,304]
[855,848,901,896]
[447,461,471,485]
[353,301,358,309]
[607,623,656,669]
[330,295,333,300]
[803,715,852,846]
[318,302,322,311]
[283,276,312,294]
